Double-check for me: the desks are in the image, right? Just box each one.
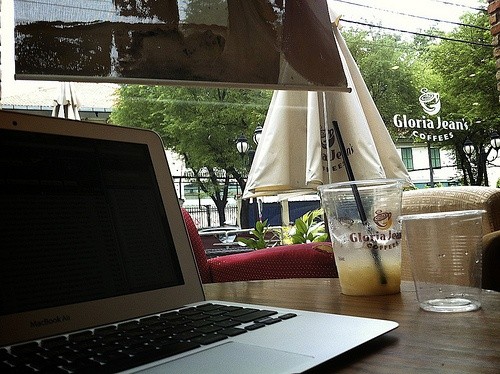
[202,278,500,374]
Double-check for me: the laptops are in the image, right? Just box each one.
[0,110,400,374]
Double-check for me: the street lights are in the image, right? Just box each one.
[236,122,263,168]
[461,132,500,186]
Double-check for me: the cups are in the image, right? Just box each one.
[317,178,407,296]
[396,210,489,313]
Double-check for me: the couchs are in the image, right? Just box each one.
[182,208,338,283]
[402,185,500,293]
